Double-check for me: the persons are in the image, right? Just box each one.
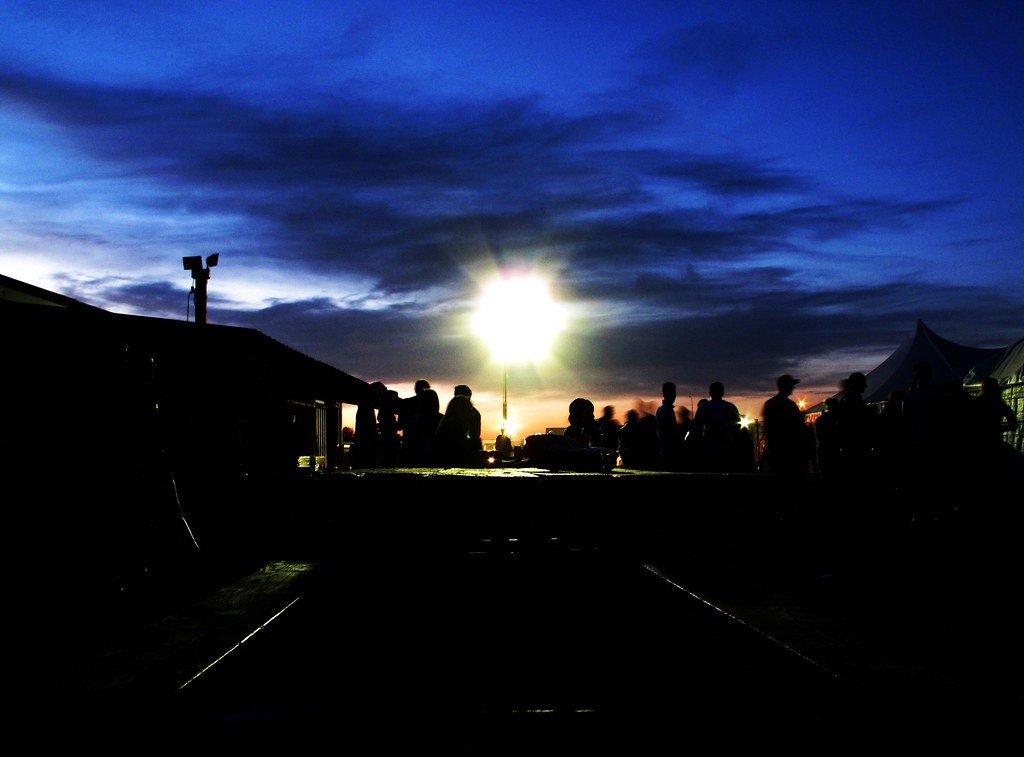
[354,361,1018,472]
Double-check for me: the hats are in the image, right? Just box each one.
[455,386,471,397]
[777,375,801,388]
